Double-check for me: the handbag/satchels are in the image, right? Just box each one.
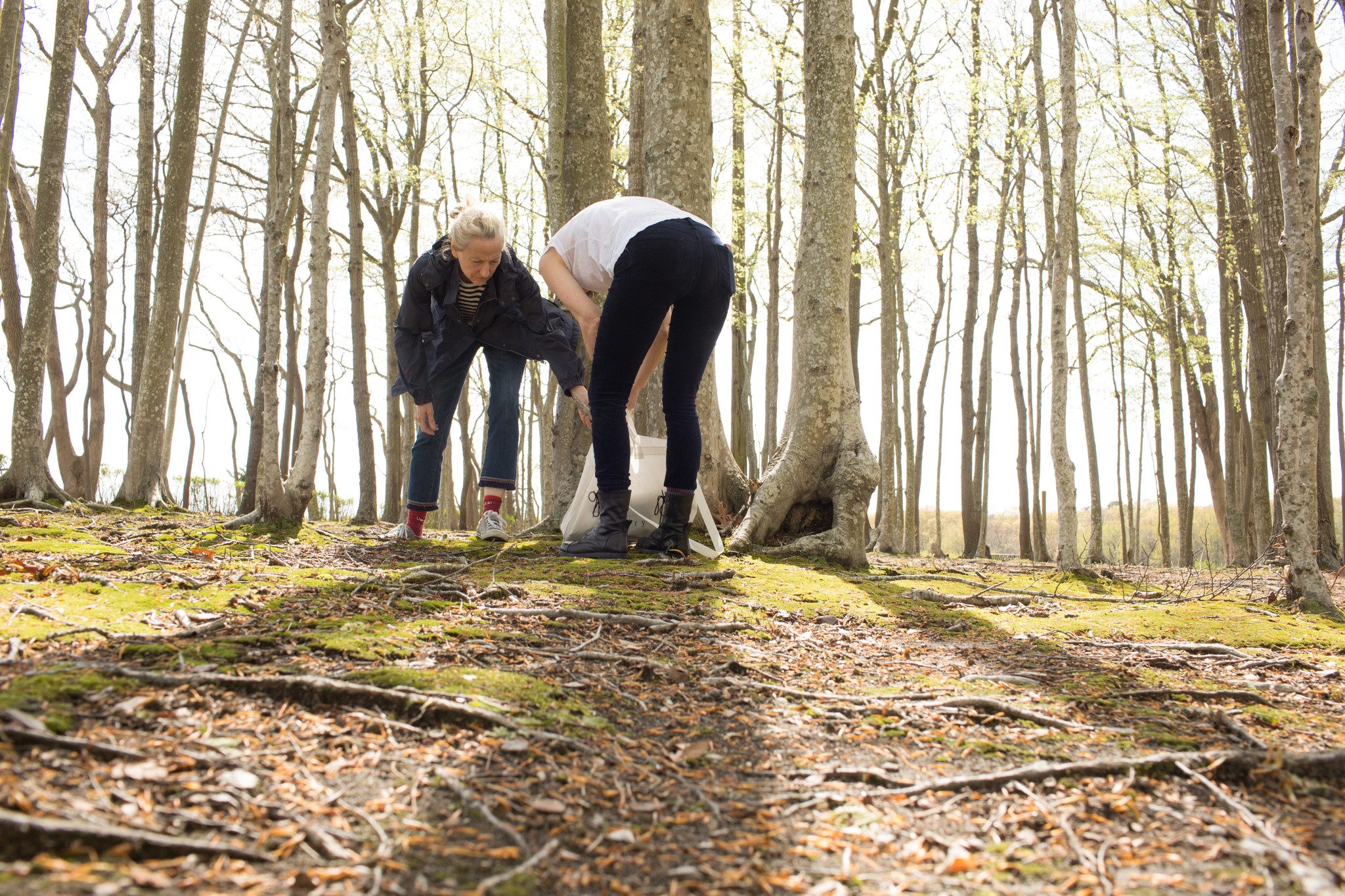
[560,408,724,559]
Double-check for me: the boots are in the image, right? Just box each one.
[559,490,633,558]
[635,490,695,558]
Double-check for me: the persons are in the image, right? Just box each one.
[538,195,736,561]
[379,201,592,543]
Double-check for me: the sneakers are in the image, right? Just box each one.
[476,510,509,541]
[380,523,423,539]
